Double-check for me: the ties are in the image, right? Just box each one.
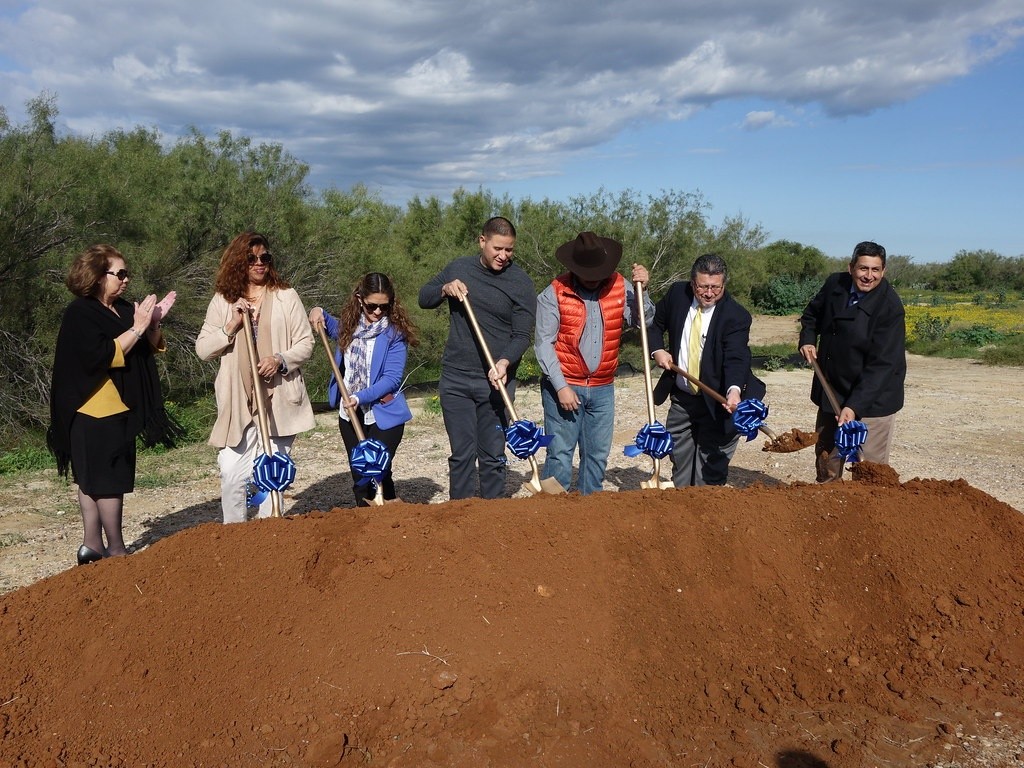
[687,305,702,394]
[849,292,858,303]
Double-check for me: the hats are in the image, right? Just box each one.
[556,231,622,281]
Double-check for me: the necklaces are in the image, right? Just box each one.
[246,297,259,304]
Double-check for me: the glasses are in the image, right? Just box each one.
[248,253,273,264]
[105,269,129,280]
[361,298,391,313]
[694,281,723,292]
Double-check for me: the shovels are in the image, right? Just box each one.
[317,321,403,505]
[667,361,819,453]
[458,289,568,495]
[809,350,866,471]
[632,263,675,490]
[242,308,283,517]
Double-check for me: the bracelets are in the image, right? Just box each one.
[220,326,235,338]
[130,325,142,338]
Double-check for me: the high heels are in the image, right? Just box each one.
[77,545,109,567]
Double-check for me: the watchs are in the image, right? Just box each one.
[147,322,161,331]
[271,355,280,366]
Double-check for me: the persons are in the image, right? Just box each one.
[196,232,316,525]
[416,217,537,500]
[533,230,657,494]
[798,241,906,484]
[646,252,766,486]
[308,273,412,507]
[52,253,190,565]
